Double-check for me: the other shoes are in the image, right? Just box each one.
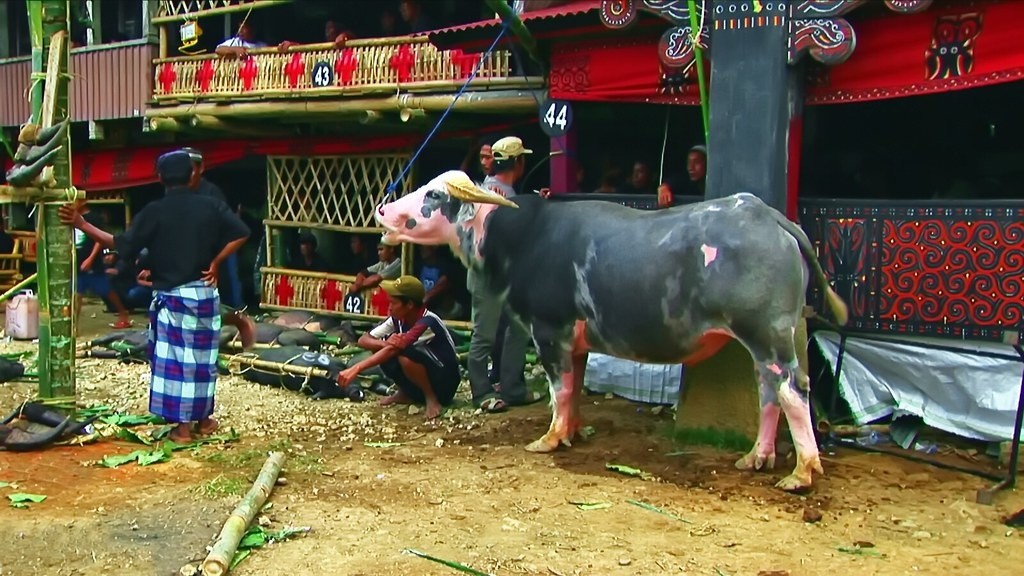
[109,320,134,328]
[236,312,257,352]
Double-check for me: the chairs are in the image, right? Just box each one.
[0,238,25,290]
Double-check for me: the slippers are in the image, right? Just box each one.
[198,419,219,434]
[168,427,192,444]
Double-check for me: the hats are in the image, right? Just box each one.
[156,150,192,179]
[492,136,533,161]
[379,276,427,302]
[182,147,203,163]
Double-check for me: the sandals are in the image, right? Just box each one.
[507,391,546,406]
[474,397,508,412]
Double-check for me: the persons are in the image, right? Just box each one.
[282,233,322,271]
[58,147,256,445]
[215,0,436,57]
[336,136,707,421]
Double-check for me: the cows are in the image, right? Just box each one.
[375,170,849,487]
